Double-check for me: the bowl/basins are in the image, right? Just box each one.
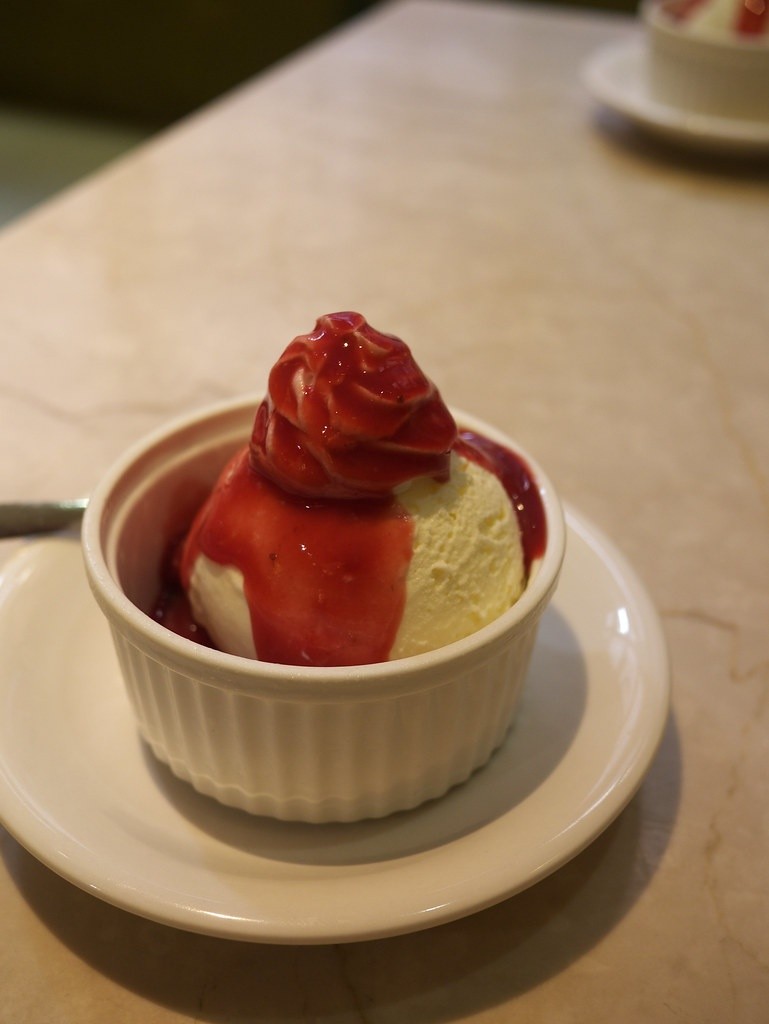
[81,388,567,826]
[642,0,769,122]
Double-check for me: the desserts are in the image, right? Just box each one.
[80,310,565,827]
[636,0,769,125]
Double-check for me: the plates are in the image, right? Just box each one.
[0,503,668,943]
[587,43,769,154]
